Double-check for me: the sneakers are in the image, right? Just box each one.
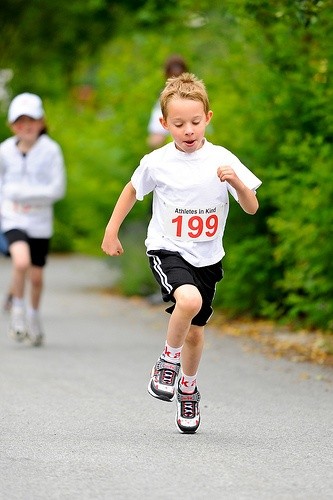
[147,354,181,402]
[174,377,201,434]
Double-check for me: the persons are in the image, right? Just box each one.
[99,70,263,436]
[0,92,67,344]
[144,58,189,148]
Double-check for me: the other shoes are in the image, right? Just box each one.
[5,298,28,342]
[24,309,44,345]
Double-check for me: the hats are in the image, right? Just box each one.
[8,93,43,123]
